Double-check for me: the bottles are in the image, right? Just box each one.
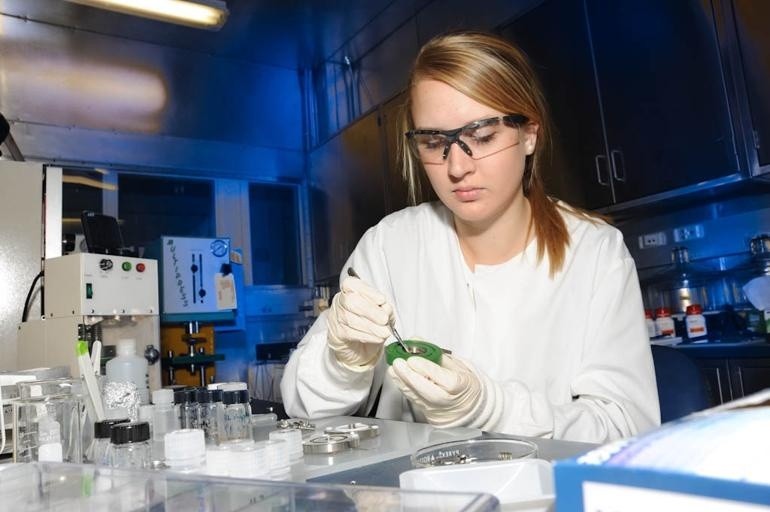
[107,339,149,407]
[89,381,304,470]
[643,305,708,338]
[12,376,82,470]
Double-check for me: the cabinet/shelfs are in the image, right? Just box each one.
[656,358,769,423]
[492,1,750,222]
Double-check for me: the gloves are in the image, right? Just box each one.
[325,277,395,366]
[387,354,482,425]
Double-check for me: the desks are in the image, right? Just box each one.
[247,416,603,486]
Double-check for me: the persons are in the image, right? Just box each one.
[280,27,661,445]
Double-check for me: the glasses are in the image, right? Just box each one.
[404,114,528,165]
